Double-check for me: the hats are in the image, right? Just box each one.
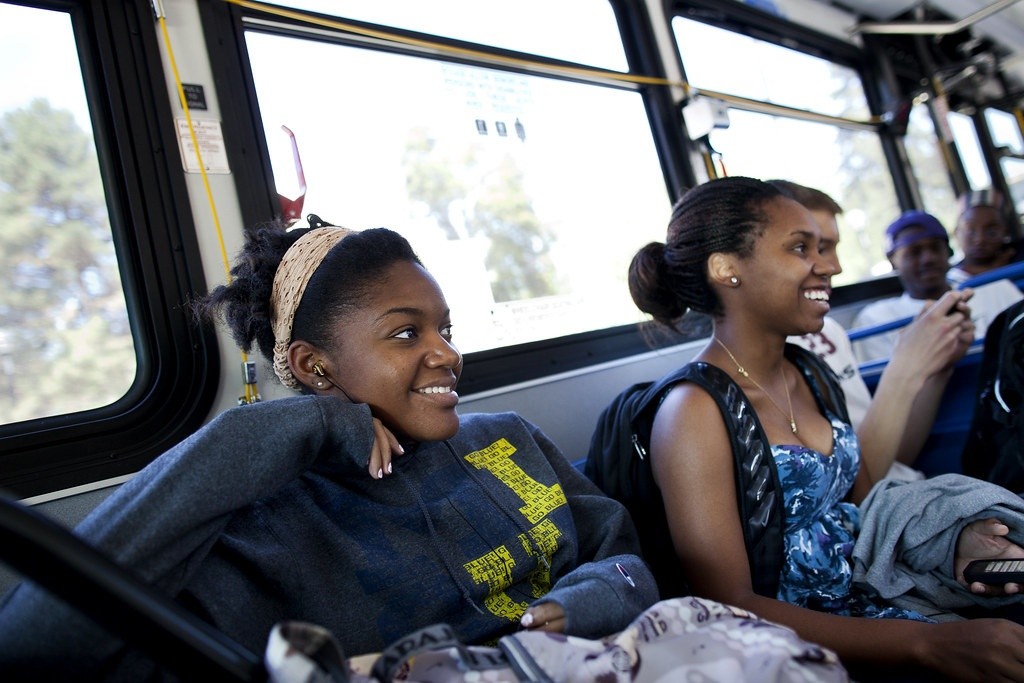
[884,211,954,258]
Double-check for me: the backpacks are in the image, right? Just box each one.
[960,295,1024,496]
[584,343,852,599]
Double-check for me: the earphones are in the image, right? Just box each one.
[311,360,326,377]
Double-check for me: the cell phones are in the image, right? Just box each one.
[963,559,1024,584]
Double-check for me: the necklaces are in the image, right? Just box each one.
[712,336,797,434]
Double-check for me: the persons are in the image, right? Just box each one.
[0,214,660,683]
[765,179,975,505]
[627,176,1024,683]
[945,190,1024,289]
[848,213,1024,362]
